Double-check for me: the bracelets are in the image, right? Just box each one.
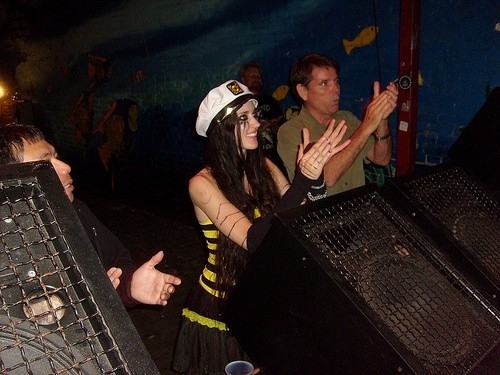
[375,131,392,143]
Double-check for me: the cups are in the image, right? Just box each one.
[225,360,254,375]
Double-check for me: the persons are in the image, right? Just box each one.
[448,86,500,207]
[175,79,352,375]
[238,61,288,177]
[276,53,398,197]
[0,43,187,321]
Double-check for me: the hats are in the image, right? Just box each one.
[195,80,259,137]
